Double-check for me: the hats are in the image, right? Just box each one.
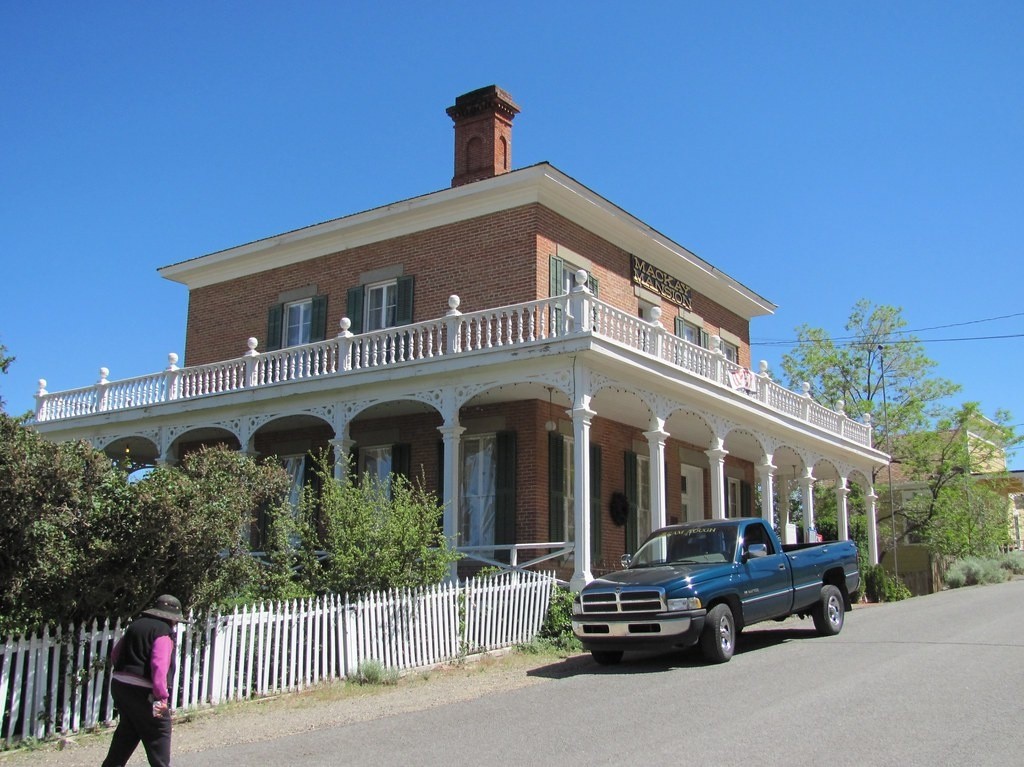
[140,594,192,624]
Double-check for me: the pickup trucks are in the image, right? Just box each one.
[571,517,860,663]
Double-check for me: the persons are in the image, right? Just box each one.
[102,595,187,767]
